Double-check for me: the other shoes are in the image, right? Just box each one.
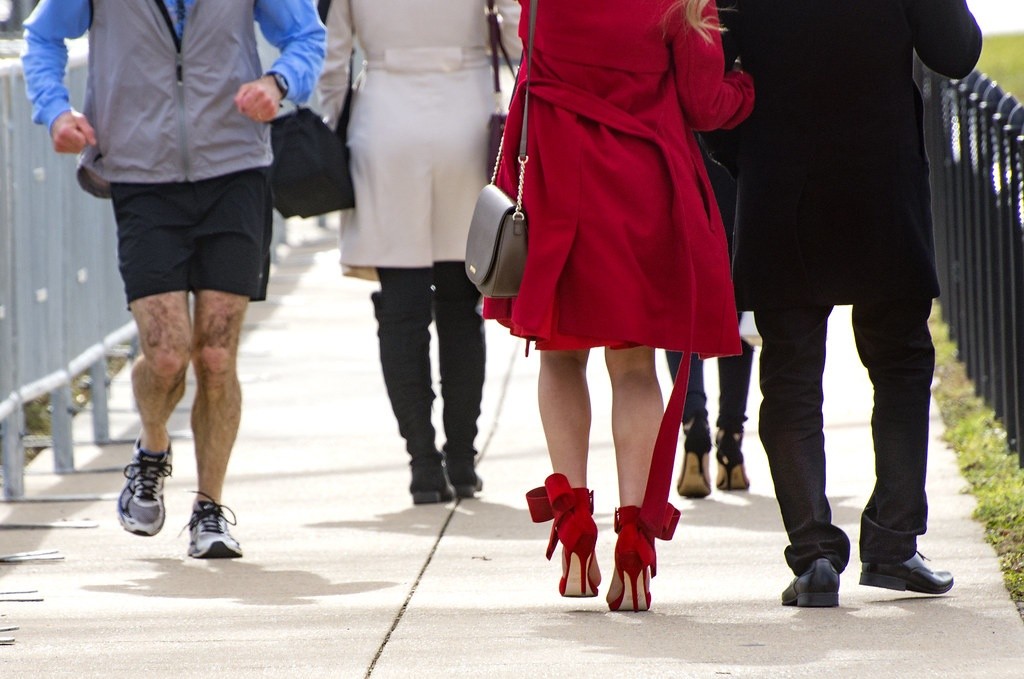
[448,465,483,497]
[410,469,457,505]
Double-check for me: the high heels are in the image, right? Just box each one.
[606,506,656,612]
[717,431,749,491]
[677,420,711,498]
[525,472,600,599]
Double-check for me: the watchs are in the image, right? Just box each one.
[268,73,288,107]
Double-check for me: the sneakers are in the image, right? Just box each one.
[117,429,173,537]
[186,489,242,558]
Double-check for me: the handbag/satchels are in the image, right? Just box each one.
[268,110,356,219]
[488,111,506,174]
[465,184,527,298]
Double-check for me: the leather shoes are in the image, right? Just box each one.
[781,557,839,610]
[859,549,954,594]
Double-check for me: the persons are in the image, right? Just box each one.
[20,0,326,558]
[315,0,524,505]
[484,0,755,611]
[666,313,754,497]
[700,0,983,607]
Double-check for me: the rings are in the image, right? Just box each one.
[257,113,262,120]
